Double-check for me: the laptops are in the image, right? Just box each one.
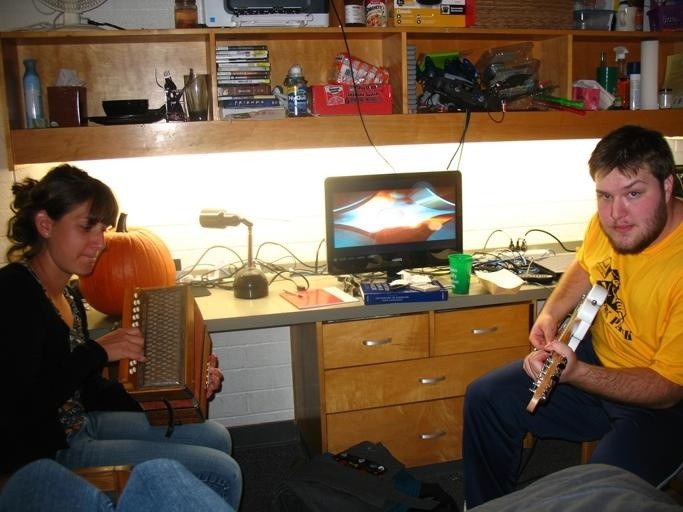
[532,253,576,279]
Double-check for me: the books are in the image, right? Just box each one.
[359,279,449,306]
[215,44,286,120]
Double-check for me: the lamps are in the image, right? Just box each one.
[200,209,267,300]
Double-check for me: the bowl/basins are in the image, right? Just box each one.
[101,98,149,118]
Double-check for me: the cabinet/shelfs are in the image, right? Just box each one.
[2,27,683,165]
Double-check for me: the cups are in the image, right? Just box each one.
[447,253,473,295]
[616,6,637,32]
[182,73,209,123]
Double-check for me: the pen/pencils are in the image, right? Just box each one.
[282,287,304,297]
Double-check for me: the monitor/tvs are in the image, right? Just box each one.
[324,170,463,285]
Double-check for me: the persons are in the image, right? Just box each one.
[462,124,683,512]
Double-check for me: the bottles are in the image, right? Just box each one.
[614,53,630,111]
[173,0,198,29]
[284,65,310,119]
[658,87,673,111]
[162,71,181,113]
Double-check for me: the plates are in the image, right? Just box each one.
[84,103,166,126]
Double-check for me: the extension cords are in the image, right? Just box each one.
[485,249,548,260]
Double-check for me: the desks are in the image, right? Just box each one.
[84,251,579,468]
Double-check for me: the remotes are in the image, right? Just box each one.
[518,273,553,283]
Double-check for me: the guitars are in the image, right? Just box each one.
[526,283,610,414]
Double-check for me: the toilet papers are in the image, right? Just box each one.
[640,39,661,110]
